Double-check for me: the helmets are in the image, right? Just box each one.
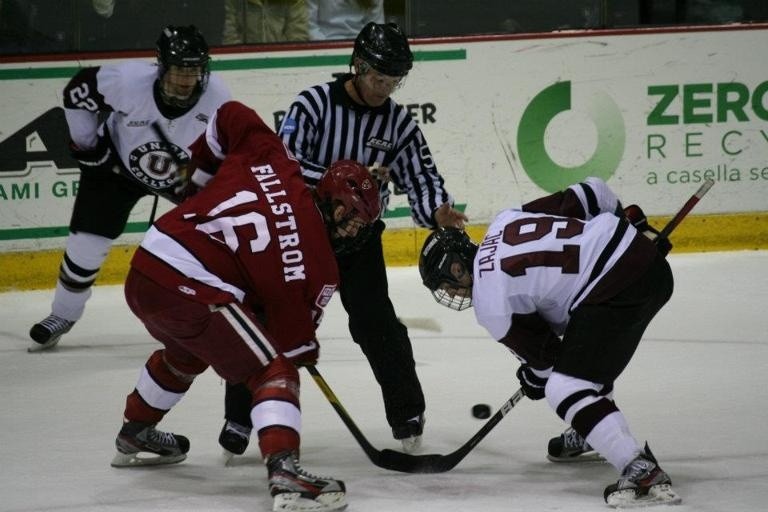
[155,24,210,108]
[318,160,380,238]
[352,22,412,92]
[419,227,480,310]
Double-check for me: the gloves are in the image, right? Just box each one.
[516,364,548,400]
[74,145,106,179]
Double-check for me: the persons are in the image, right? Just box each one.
[116,102,382,497]
[221,2,311,44]
[30,26,227,348]
[418,177,674,507]
[310,0,384,42]
[219,22,467,455]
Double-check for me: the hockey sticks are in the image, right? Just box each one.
[379,177,715,472]
[151,122,445,470]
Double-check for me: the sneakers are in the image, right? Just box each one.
[548,431,594,457]
[30,315,74,343]
[219,421,251,454]
[604,454,671,503]
[392,413,424,439]
[115,417,189,456]
[265,449,345,499]
